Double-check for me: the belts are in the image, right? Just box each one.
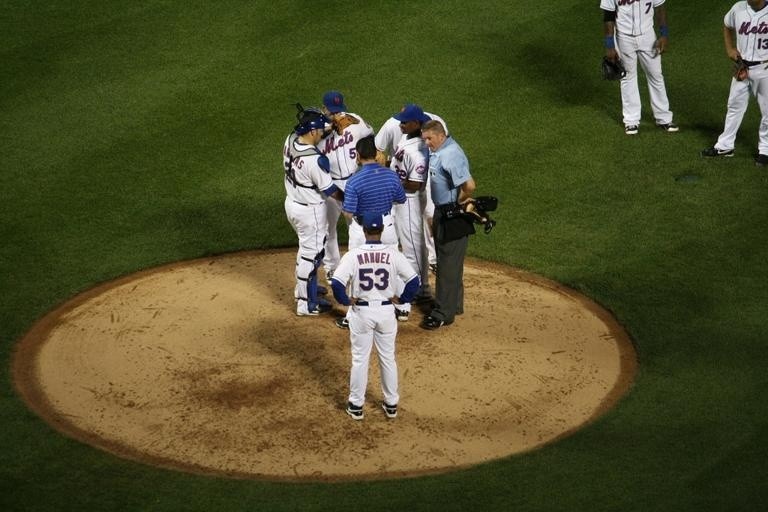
[742,60,768,67]
[354,300,393,306]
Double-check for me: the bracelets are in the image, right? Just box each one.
[604,36,615,49]
[657,24,669,40]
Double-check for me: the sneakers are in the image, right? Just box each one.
[395,306,408,321]
[382,399,398,419]
[701,144,734,158]
[624,124,638,135]
[317,285,328,297]
[336,315,349,329]
[754,149,768,167]
[416,296,434,315]
[345,398,365,421]
[319,298,333,315]
[656,117,680,133]
[420,314,454,329]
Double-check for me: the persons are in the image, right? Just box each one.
[696,1,768,170]
[598,1,679,137]
[328,213,419,422]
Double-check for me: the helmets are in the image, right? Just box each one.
[294,108,331,136]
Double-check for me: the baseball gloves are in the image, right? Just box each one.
[332,112,359,135]
[730,55,747,81]
[603,55,626,82]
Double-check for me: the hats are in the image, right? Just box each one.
[393,105,426,122]
[322,91,346,113]
[361,210,383,228]
